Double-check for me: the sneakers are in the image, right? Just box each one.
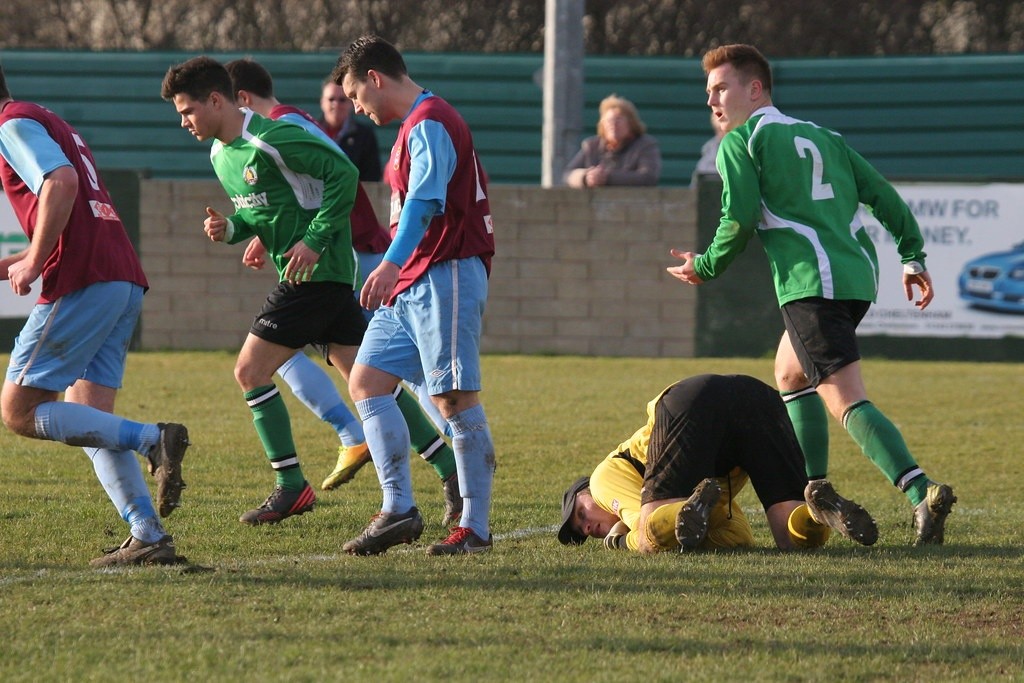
[343,506,426,554]
[440,471,464,529]
[146,423,191,518]
[89,533,175,568]
[912,481,958,549]
[240,479,317,525]
[321,441,371,491]
[806,481,879,545]
[676,478,723,547]
[426,525,493,557]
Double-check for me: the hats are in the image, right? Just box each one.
[556,474,591,547]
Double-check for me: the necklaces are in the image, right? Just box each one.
[1,100,13,114]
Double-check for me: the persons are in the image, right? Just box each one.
[555,372,879,553]
[667,44,959,548]
[159,55,467,528]
[218,58,456,487]
[332,32,500,556]
[312,77,387,182]
[560,93,729,189]
[0,73,192,567]
